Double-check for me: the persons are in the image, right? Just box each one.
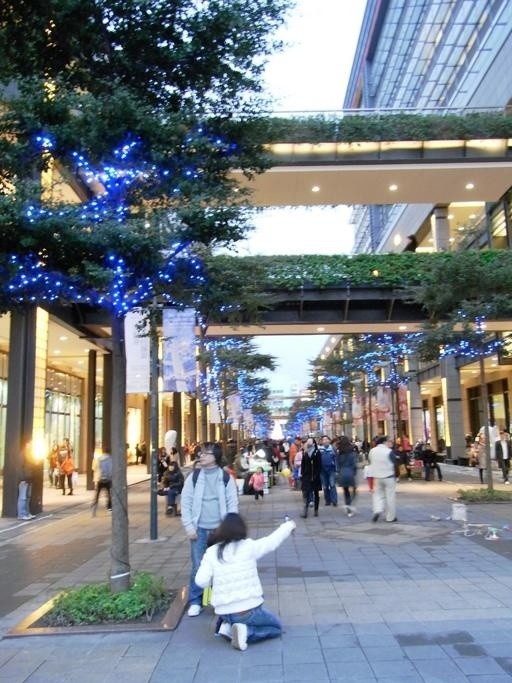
[400,234,419,253]
[18,437,41,520]
[464,419,511,485]
[195,511,297,650]
[179,443,240,618]
[91,445,112,518]
[46,438,74,495]
[135,433,445,522]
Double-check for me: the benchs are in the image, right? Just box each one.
[165,494,181,516]
[407,465,434,481]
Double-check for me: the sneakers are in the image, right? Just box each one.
[17,514,35,520]
[188,605,204,616]
[232,623,247,651]
[219,623,232,640]
